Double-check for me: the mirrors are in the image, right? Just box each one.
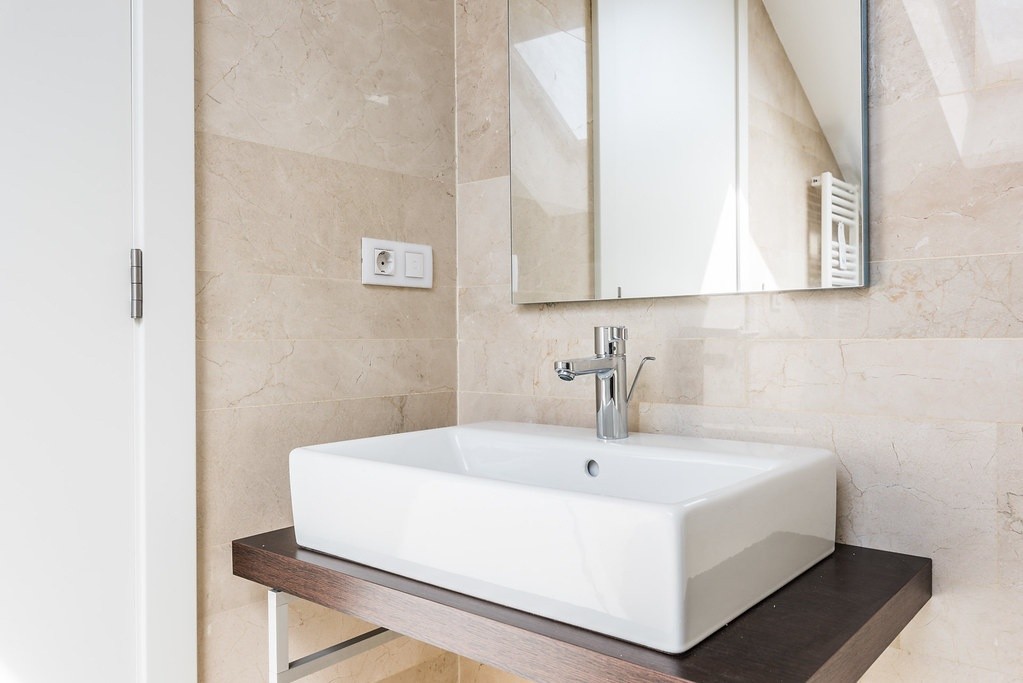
[506,0,871,304]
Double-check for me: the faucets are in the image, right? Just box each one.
[289,421,836,655]
[554,325,655,440]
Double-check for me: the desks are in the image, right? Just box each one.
[232,525,933,683]
